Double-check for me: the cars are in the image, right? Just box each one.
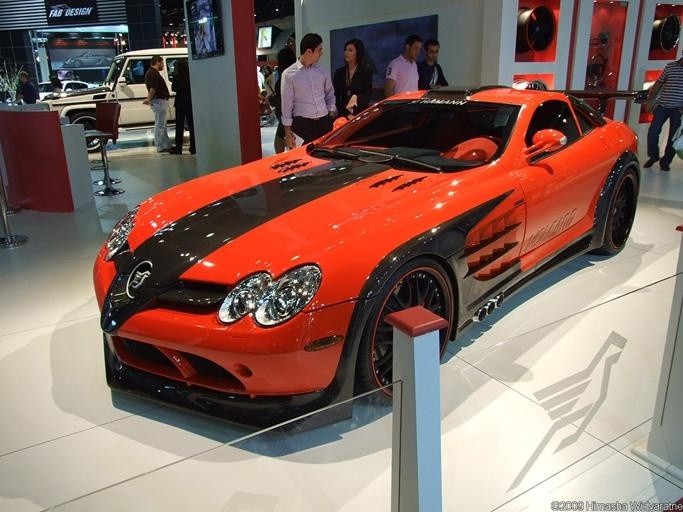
[6,80,95,107]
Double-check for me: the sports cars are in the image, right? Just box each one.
[95,81,651,446]
[65,52,116,69]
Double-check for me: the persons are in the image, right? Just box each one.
[168,58,195,154]
[585,54,607,91]
[189,1,216,56]
[256,32,449,155]
[141,56,175,153]
[49,75,62,95]
[642,51,683,170]
[11,71,37,105]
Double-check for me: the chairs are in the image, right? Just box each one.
[85,103,121,185]
[85,104,125,196]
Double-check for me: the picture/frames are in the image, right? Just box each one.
[186,0,224,60]
[257,25,274,49]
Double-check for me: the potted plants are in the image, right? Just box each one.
[0,61,24,106]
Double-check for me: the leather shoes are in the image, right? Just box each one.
[159,145,195,154]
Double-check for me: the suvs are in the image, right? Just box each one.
[36,47,187,153]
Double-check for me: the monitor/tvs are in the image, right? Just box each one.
[257,25,282,49]
